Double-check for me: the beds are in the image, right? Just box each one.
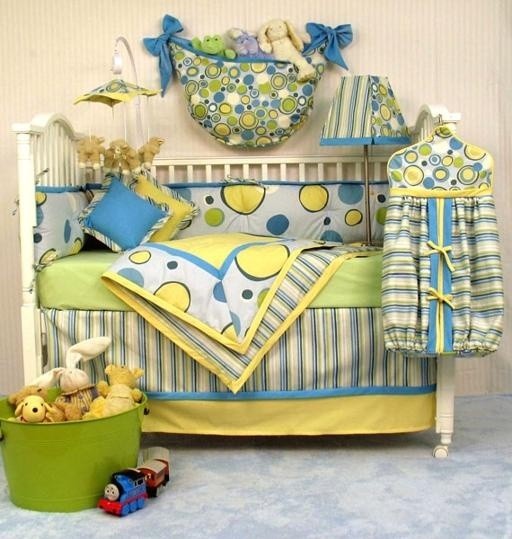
[12,105,480,458]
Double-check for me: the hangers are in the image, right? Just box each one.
[438,114,444,126]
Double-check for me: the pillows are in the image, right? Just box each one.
[126,168,200,242]
[78,173,172,252]
[30,190,88,267]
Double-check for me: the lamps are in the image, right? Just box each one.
[111,34,145,147]
[320,75,412,246]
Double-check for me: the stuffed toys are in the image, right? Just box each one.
[256,17,317,83]
[4,334,144,426]
[193,33,235,63]
[224,29,261,59]
